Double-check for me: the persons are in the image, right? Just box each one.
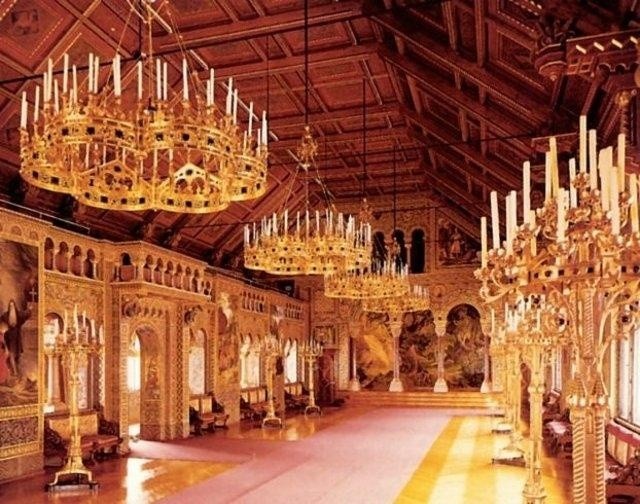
[0,300,34,385]
[442,225,476,260]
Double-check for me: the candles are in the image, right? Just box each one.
[259,335,281,351]
[46,303,106,345]
[481,115,640,338]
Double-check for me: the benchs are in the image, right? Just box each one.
[522,389,640,504]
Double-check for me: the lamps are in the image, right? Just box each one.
[303,339,323,353]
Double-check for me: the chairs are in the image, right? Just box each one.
[43,405,123,468]
[239,386,281,422]
[189,393,230,436]
[285,382,310,412]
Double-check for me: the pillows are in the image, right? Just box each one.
[200,396,212,414]
[78,414,98,437]
[249,391,258,404]
[284,386,290,395]
[189,399,200,412]
[290,386,296,395]
[258,389,266,403]
[49,418,71,442]
[296,385,302,396]
[241,391,248,403]
